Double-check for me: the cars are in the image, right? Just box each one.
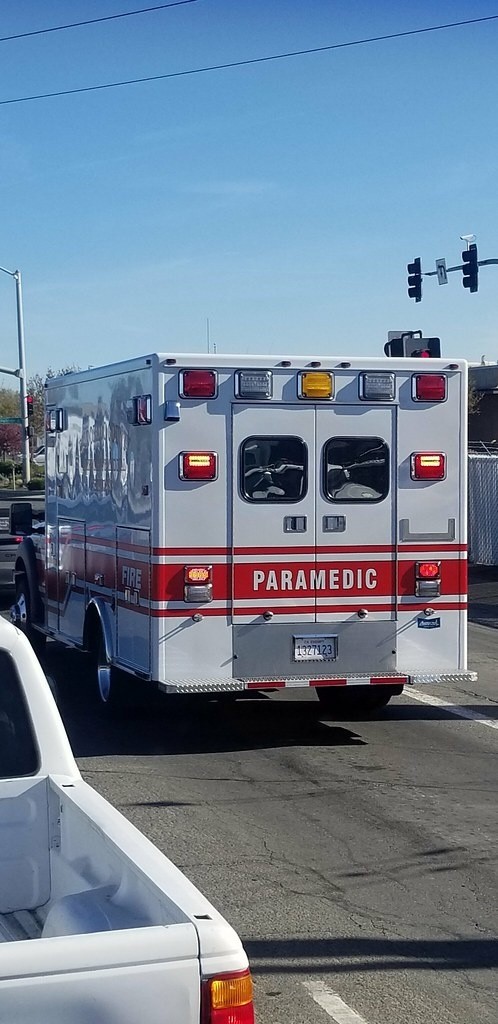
[18,444,45,466]
[0,506,32,598]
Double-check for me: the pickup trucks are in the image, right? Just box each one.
[0,616,254,1024]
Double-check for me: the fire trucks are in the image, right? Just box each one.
[6,347,468,720]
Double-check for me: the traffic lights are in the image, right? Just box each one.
[407,257,422,302]
[26,396,34,413]
[405,335,443,360]
[462,243,477,292]
